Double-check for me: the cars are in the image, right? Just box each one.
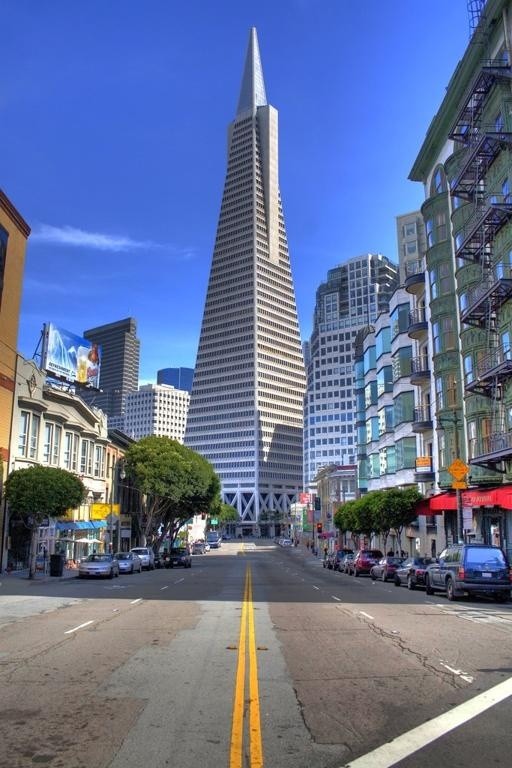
[222,534,230,540]
[322,542,437,591]
[78,547,192,578]
[274,536,293,548]
[192,541,210,555]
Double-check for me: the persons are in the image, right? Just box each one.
[306,540,310,550]
[323,544,328,559]
[387,548,394,557]
[311,540,314,549]
[93,550,96,560]
[396,548,404,557]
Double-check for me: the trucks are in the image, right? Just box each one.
[207,532,222,549]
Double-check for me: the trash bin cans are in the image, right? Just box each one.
[49,554,65,576]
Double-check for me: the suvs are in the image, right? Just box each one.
[423,543,512,601]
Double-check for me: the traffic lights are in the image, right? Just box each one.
[317,523,323,533]
[202,512,206,520]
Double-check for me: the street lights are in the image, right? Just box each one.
[306,501,316,549]
[109,454,126,552]
[434,411,467,542]
[334,489,347,549]
